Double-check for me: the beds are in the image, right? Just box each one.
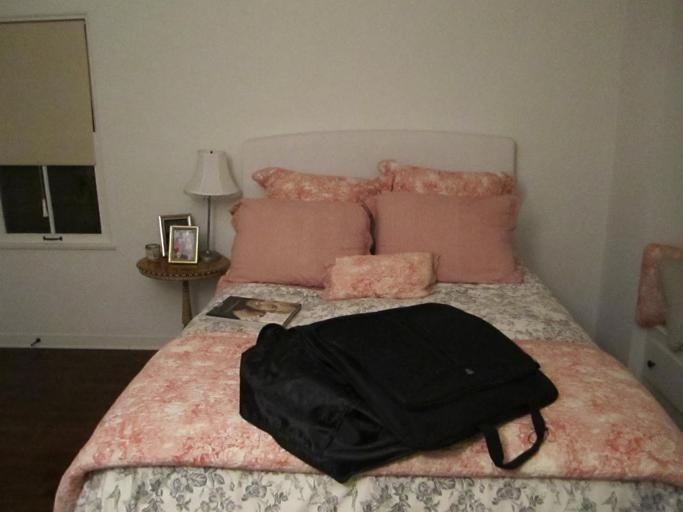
[52,128,683,512]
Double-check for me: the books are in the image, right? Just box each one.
[205,294,302,328]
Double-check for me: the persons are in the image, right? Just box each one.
[230,307,291,325]
[243,299,294,314]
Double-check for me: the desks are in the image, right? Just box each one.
[136,255,233,327]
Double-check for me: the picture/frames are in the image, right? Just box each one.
[158,215,201,265]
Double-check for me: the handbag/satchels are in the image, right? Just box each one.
[238,303,558,483]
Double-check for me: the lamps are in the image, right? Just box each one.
[181,148,237,260]
